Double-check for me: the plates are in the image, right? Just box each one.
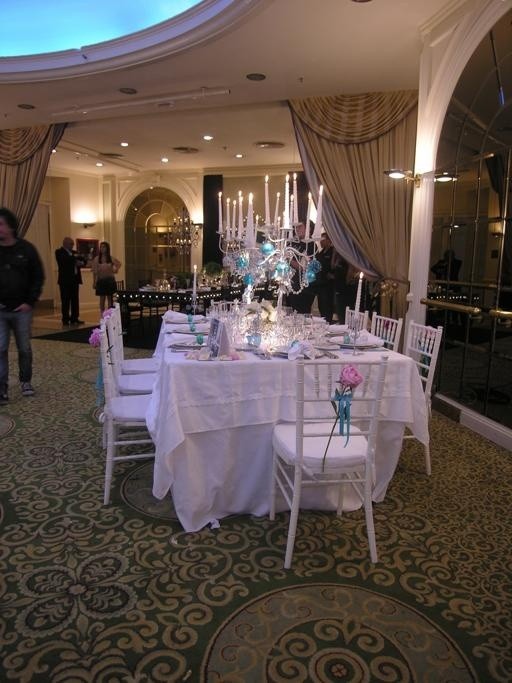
[166,314,382,358]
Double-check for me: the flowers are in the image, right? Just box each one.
[88,327,101,347]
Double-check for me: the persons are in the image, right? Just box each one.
[54,236,89,327]
[429,246,464,335]
[283,219,380,326]
[1,206,46,406]
[90,242,122,316]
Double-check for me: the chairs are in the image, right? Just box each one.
[101,298,157,507]
[114,279,169,328]
[210,298,241,312]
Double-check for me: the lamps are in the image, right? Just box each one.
[434,171,461,184]
[382,167,422,188]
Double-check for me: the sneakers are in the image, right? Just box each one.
[0,384,9,405]
[19,383,34,396]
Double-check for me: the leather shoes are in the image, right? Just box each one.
[70,320,84,324]
[63,320,69,325]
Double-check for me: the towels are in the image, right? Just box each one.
[163,308,213,345]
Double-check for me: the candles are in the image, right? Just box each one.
[354,278,362,316]
[218,174,325,242]
[193,268,197,293]
